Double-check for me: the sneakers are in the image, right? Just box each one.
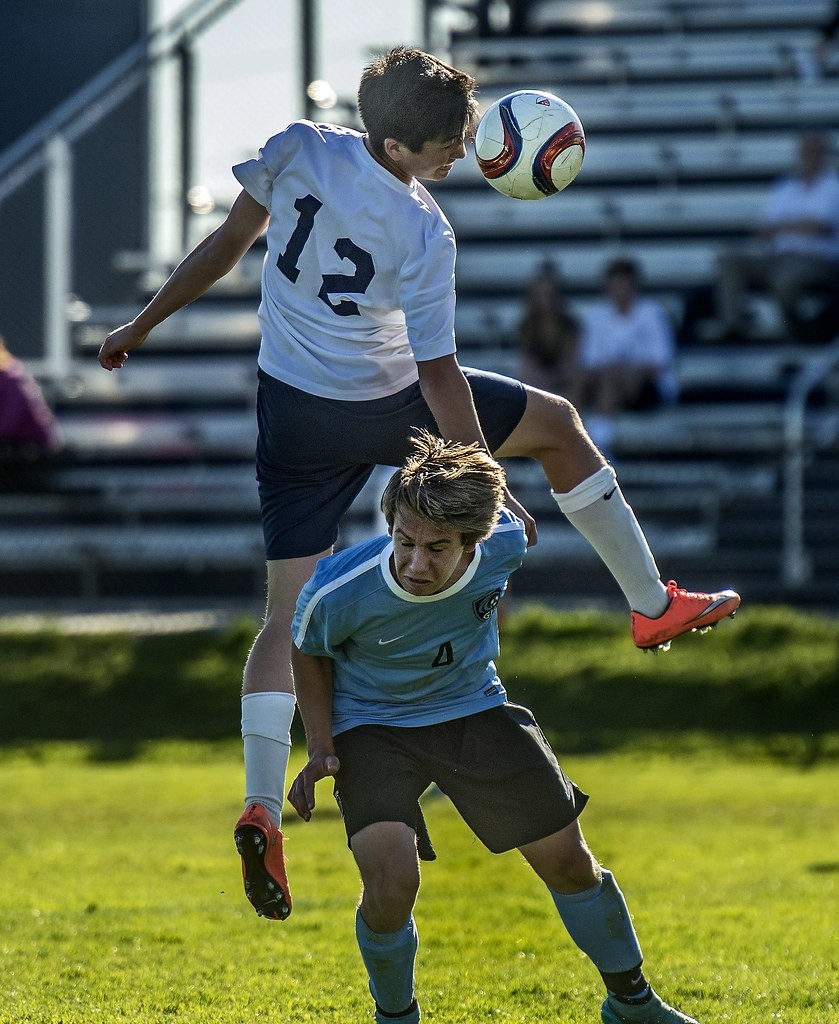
[630,578,740,657]
[233,802,292,921]
[601,997,699,1024]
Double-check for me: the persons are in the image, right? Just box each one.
[703,134,839,344]
[504,255,683,443]
[98,45,740,921]
[287,426,703,1024]
[0,342,92,495]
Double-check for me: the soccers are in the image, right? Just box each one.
[475,89,587,200]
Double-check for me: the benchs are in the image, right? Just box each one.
[0,0,839,598]
